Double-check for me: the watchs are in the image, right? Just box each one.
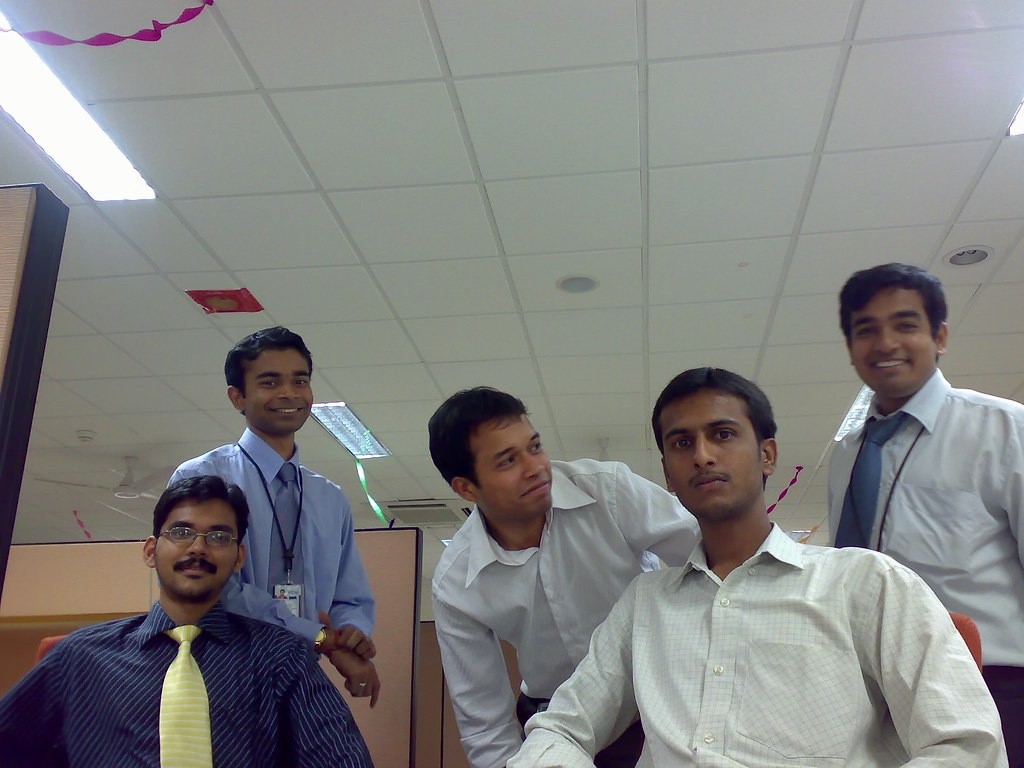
[313,629,327,655]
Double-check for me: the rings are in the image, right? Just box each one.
[359,682,368,686]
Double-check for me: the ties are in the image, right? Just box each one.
[267,465,301,617]
[834,411,907,548]
[159,625,213,768]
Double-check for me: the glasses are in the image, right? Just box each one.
[156,527,238,546]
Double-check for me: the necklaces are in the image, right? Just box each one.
[850,426,924,552]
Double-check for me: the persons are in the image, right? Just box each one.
[428,385,703,768]
[826,263,1024,768]
[164,326,382,708]
[505,367,1009,768]
[277,588,287,600]
[0,475,376,768]
